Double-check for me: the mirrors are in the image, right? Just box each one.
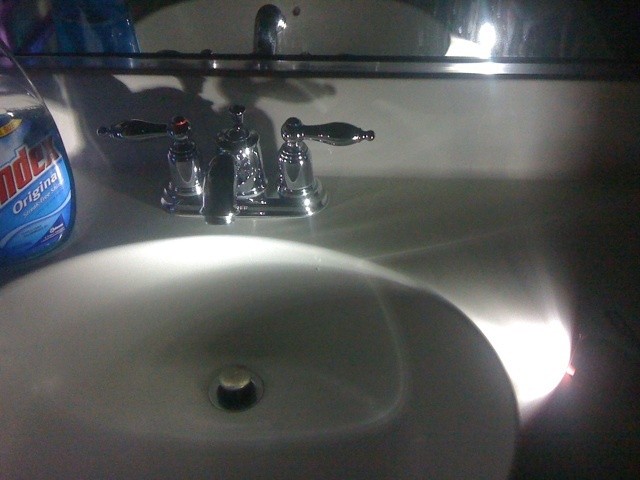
[2,0,639,85]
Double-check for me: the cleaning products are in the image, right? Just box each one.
[18,5,140,70]
[1,42,76,265]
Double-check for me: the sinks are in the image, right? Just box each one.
[0,235,520,480]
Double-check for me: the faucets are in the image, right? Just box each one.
[199,102,271,226]
[248,4,289,72]
